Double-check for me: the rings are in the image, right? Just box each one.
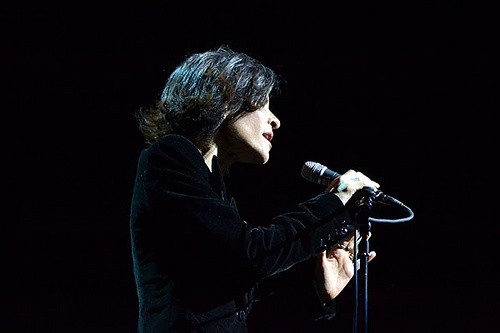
[346,245,351,251]
[338,243,346,249]
[349,253,354,260]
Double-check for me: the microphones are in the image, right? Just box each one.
[301,161,410,212]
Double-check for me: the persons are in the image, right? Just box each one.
[129,47,380,333]
